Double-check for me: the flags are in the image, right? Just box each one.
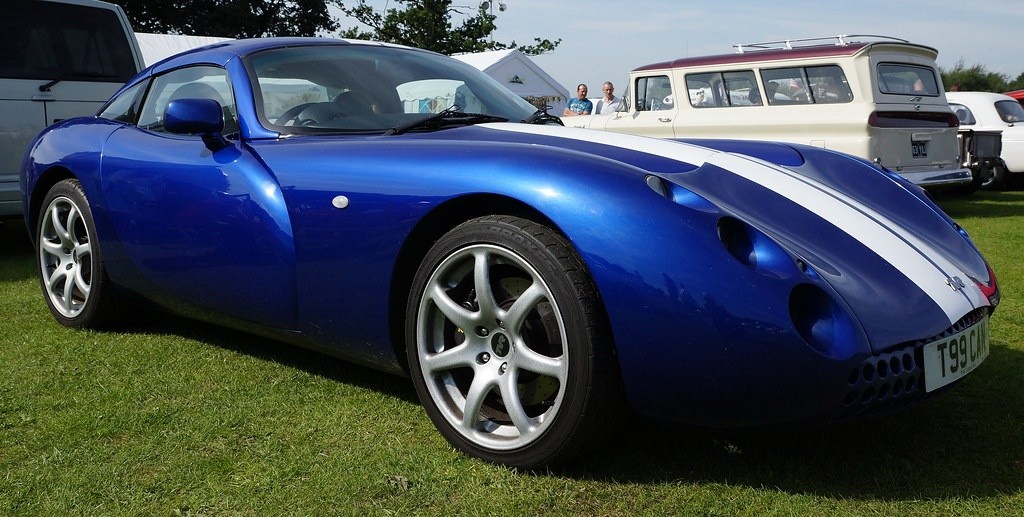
[789,77,800,89]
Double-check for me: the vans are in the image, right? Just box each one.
[0,0,149,214]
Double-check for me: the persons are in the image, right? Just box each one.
[563,83,593,117]
[595,81,626,113]
[912,78,960,94]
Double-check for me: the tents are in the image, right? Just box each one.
[132,32,330,130]
[393,46,572,121]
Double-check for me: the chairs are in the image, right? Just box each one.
[163,82,239,135]
[331,90,374,117]
[955,109,968,125]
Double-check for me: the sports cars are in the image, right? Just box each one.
[21,34,1004,468]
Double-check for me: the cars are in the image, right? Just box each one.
[944,91,1024,189]
[556,33,976,188]
